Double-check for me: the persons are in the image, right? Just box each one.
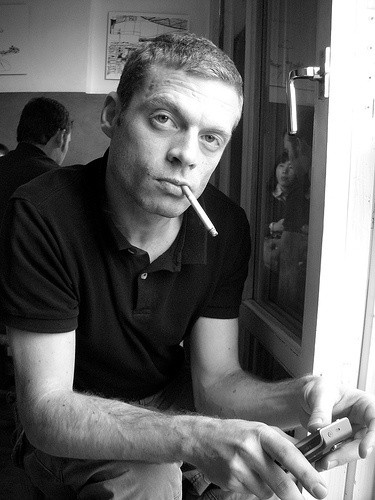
[0,32,375,500]
[262,129,313,313]
[0,96,74,215]
[266,151,294,238]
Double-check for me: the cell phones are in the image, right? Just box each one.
[274,417,352,473]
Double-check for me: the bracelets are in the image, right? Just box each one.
[270,222,276,234]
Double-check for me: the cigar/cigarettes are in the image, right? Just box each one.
[181,184,218,237]
